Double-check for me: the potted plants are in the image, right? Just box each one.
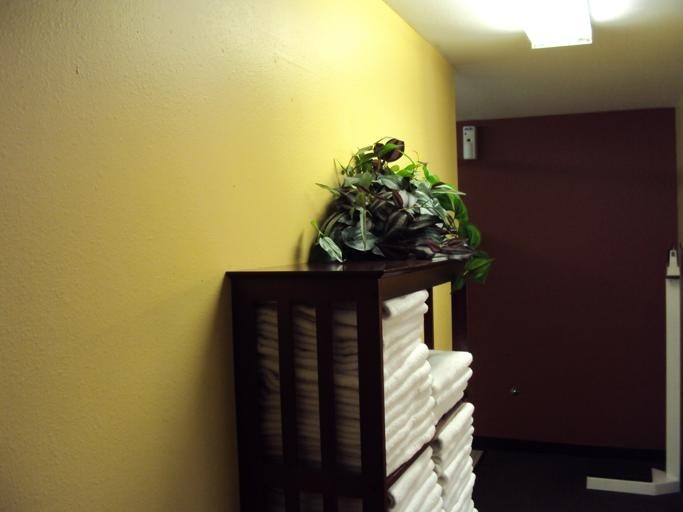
[306,140,495,295]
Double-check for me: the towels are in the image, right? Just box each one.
[258,288,480,512]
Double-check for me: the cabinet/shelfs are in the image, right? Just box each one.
[223,254,466,511]
